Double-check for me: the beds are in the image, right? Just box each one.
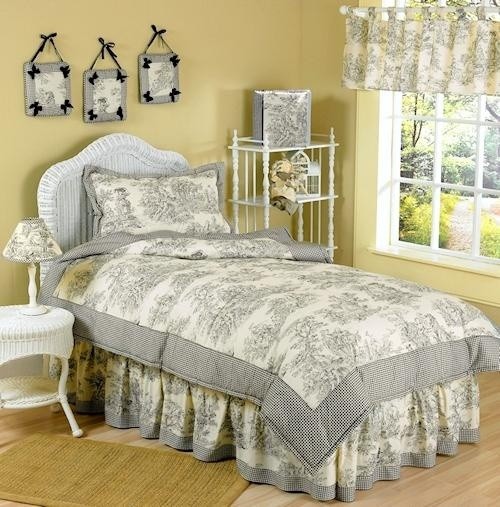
[37,132,498,502]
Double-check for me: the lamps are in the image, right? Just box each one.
[1,219,64,317]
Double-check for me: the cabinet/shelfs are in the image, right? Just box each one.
[233,126,340,255]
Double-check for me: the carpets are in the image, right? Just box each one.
[1,433,253,507]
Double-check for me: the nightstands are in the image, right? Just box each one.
[0,304,83,437]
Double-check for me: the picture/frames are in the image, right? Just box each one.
[24,62,72,116]
[139,52,179,105]
[82,67,127,124]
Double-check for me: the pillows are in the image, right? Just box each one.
[84,160,232,240]
